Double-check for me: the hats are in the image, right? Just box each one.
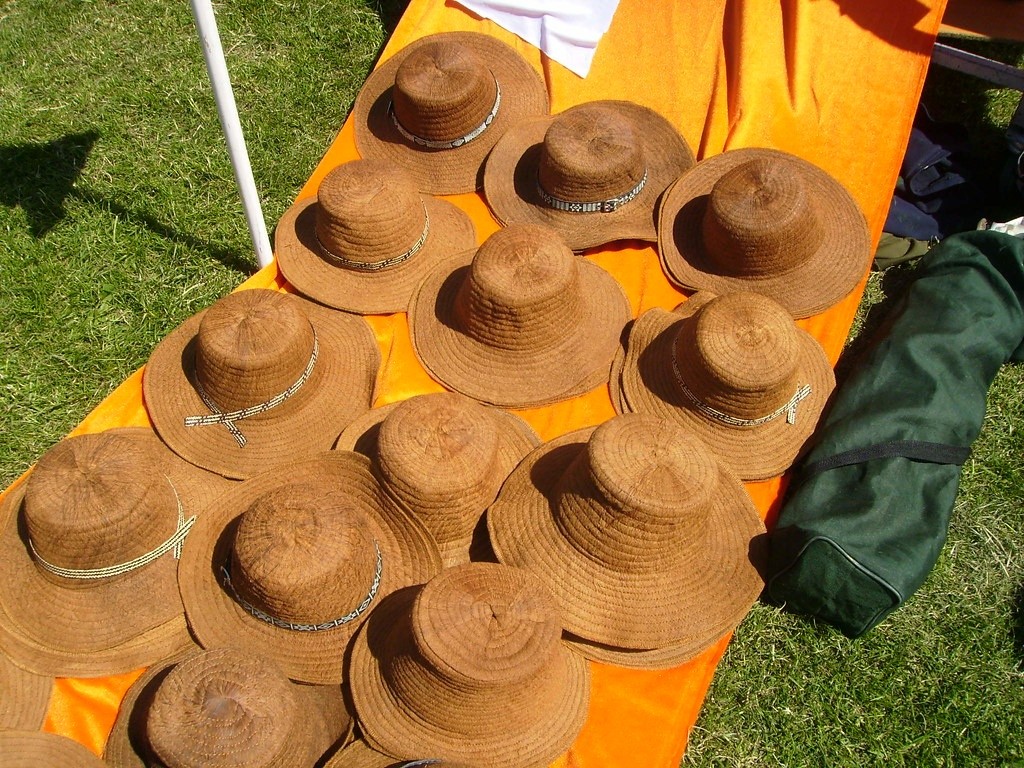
[3,27,870,768]
